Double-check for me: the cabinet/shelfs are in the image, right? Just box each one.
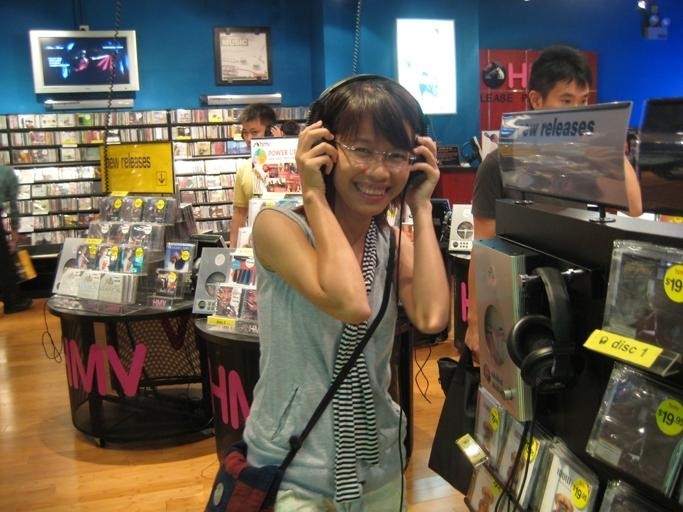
[0,107,310,298]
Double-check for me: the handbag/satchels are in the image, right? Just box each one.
[9,245,38,285]
[204,220,397,510]
[427,339,480,497]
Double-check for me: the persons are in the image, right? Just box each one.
[243,75,451,512]
[217,287,257,321]
[230,103,284,248]
[623,132,641,172]
[78,247,132,274]
[280,119,301,137]
[464,45,644,366]
[0,162,33,312]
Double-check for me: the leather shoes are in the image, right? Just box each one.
[4,297,32,314]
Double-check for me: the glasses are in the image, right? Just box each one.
[328,136,417,172]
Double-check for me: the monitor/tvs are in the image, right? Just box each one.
[29,30,140,94]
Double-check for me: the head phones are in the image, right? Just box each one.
[306,74,426,175]
[508,267,582,392]
[241,106,277,138]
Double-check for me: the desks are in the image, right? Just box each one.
[44,291,213,450]
[192,313,415,511]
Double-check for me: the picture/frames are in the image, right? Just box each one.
[212,24,272,87]
